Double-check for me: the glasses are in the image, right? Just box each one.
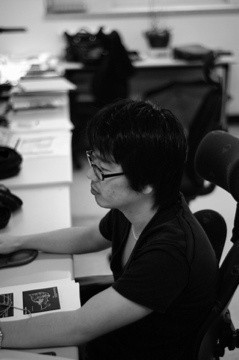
[86,150,125,181]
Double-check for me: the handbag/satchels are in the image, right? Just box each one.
[64,28,101,62]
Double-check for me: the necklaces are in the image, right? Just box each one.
[131,225,140,242]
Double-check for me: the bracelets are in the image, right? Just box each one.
[0,330,4,347]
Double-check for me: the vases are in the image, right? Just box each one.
[146,30,169,47]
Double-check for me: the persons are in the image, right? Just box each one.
[0,100,221,360]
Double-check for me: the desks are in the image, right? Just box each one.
[0,53,238,360]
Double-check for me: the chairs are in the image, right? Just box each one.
[174,131,239,360]
[64,27,138,169]
[142,79,230,199]
